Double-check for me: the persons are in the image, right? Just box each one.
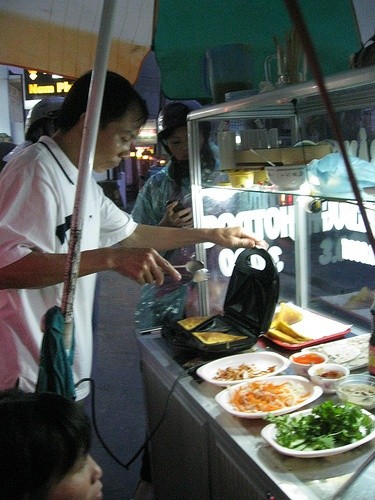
[133,99,221,334]
[0,68,265,411]
[0,95,67,173]
[0,387,102,500]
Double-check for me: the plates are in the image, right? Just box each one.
[260,404,375,459]
[195,350,291,387]
[214,374,324,419]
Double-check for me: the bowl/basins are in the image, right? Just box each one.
[332,373,375,411]
[265,165,307,191]
[308,362,350,394]
[288,352,328,377]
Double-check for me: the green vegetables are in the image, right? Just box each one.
[262,399,375,452]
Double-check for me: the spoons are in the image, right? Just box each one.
[173,260,205,273]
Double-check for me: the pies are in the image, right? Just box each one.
[192,332,249,344]
[176,317,210,331]
[268,302,313,344]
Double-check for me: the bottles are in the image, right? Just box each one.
[367,310,375,376]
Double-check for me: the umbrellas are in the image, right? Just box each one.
[0,1,372,402]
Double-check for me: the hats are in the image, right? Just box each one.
[0,133,17,148]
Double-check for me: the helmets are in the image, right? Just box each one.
[25,96,65,140]
[156,101,210,139]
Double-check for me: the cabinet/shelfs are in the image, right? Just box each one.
[136,69,375,500]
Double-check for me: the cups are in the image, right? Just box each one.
[264,31,307,88]
